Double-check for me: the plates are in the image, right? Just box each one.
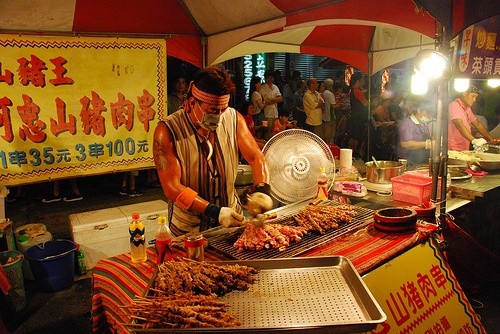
[419,167,472,180]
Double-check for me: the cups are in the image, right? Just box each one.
[339,148,353,169]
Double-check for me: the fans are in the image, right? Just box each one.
[260,128,336,205]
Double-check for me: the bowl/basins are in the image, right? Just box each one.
[364,160,406,184]
[374,206,422,234]
[235,165,254,186]
[261,121,268,127]
[447,150,475,167]
[463,150,500,170]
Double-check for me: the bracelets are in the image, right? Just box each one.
[206,201,221,220]
[254,182,272,194]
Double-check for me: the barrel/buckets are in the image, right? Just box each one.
[0,251,27,313]
[13,225,54,280]
[26,242,78,292]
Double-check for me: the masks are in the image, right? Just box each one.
[190,102,232,132]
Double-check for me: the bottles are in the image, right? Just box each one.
[317,168,328,202]
[184,233,204,262]
[399,159,407,171]
[154,216,172,265]
[128,213,147,263]
[75,244,87,276]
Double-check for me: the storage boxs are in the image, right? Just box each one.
[391,174,432,205]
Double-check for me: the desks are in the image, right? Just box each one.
[90,219,440,334]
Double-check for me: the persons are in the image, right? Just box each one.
[169,51,500,164]
[154,64,273,241]
[3,158,161,208]
